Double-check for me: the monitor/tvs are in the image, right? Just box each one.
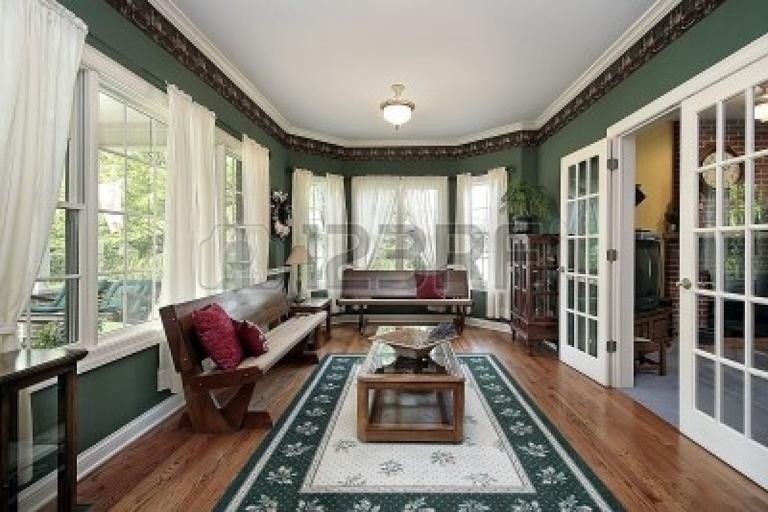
[633,238,664,312]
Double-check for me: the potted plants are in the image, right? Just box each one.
[501,184,556,231]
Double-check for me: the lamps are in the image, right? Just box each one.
[753,82,768,124]
[379,84,414,129]
[285,246,314,302]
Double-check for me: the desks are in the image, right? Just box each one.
[635,302,672,378]
[1,349,90,511]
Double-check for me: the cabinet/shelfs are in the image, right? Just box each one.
[508,233,559,356]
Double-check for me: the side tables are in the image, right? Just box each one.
[287,297,332,349]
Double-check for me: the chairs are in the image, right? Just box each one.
[21,277,108,325]
[99,278,161,326]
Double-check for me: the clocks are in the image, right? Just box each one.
[700,143,743,191]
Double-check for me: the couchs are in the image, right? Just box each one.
[337,270,472,336]
[158,279,329,434]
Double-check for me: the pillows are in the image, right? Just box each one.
[192,303,247,370]
[413,270,447,299]
[231,316,268,355]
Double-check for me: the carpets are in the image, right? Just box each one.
[208,353,629,512]
[617,347,768,449]
